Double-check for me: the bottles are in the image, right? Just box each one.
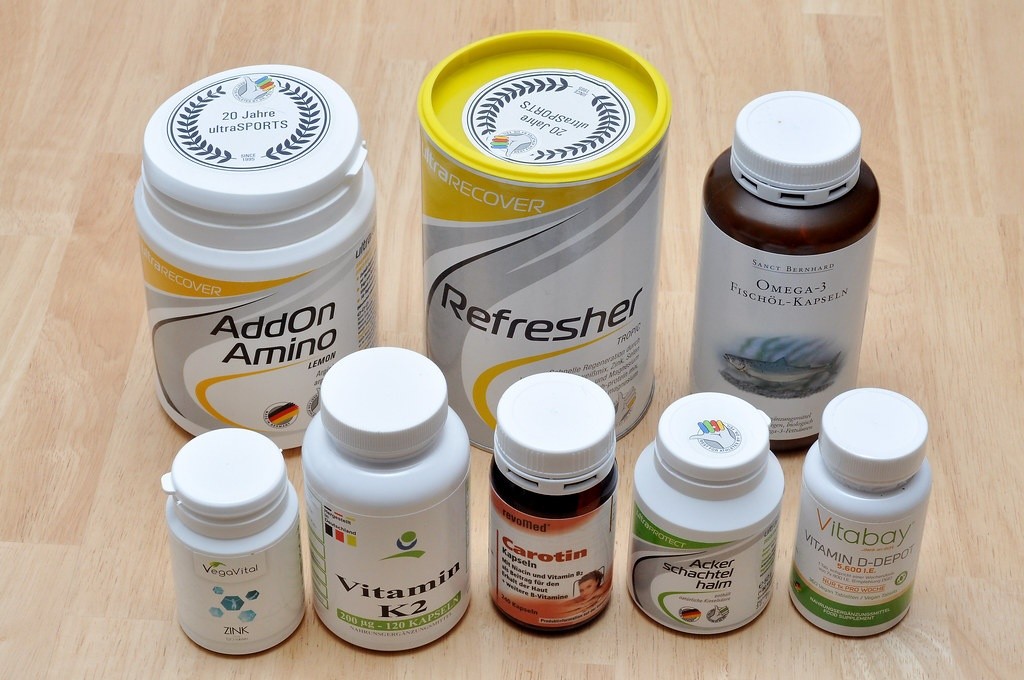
[627,391,786,637]
[300,346,473,651]
[159,426,308,657]
[789,389,931,638]
[486,370,620,635]
[419,30,672,456]
[134,64,377,450]
[689,90,881,456]
